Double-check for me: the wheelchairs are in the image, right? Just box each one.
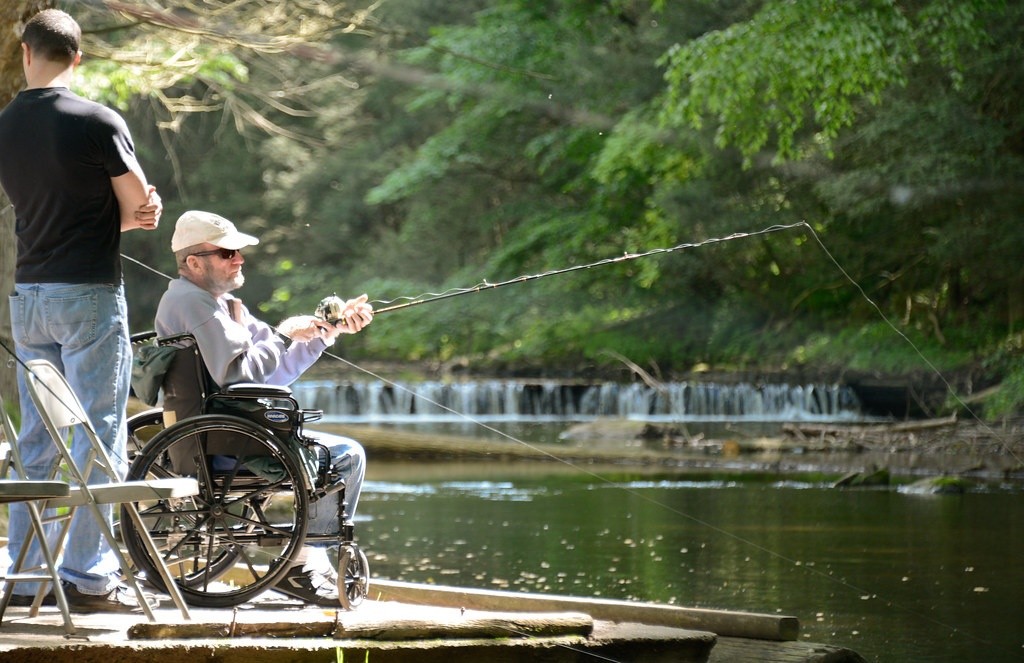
[118,330,370,607]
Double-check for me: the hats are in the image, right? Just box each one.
[171,210,260,250]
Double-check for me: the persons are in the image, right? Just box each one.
[155,210,373,608]
[0,10,163,616]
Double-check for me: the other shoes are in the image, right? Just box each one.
[7,588,58,606]
[268,558,343,608]
[320,564,357,601]
[57,581,161,612]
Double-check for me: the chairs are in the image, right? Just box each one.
[0,359,195,631]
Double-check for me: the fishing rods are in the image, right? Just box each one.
[313,218,809,329]
[0,341,197,534]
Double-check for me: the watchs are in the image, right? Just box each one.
[275,331,292,349]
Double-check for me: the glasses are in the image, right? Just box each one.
[183,248,240,263]
